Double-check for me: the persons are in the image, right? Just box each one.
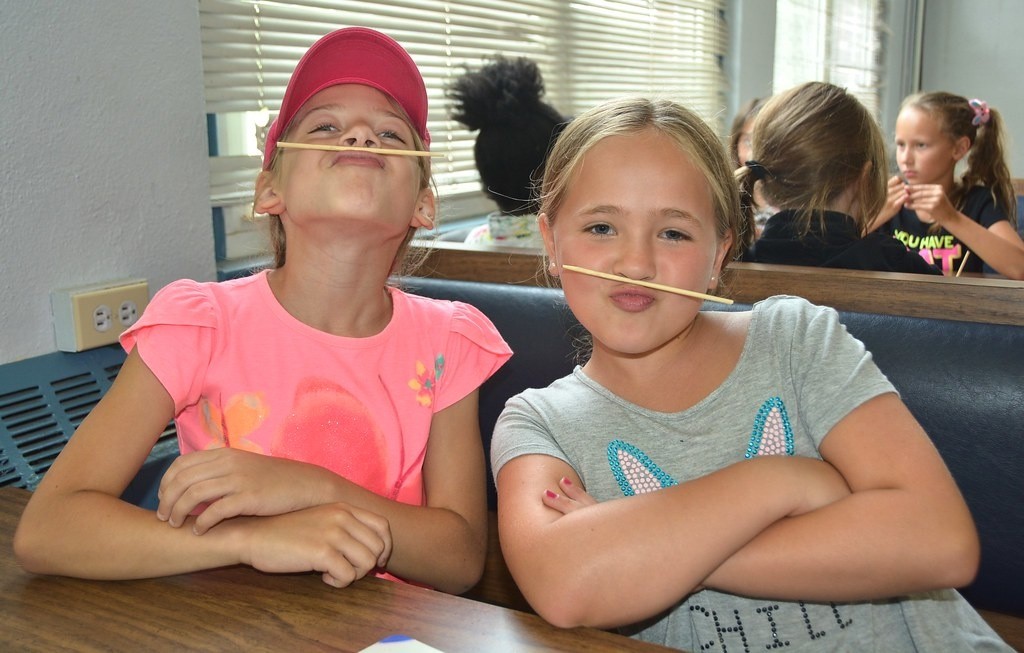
[12,27,516,596]
[488,98,1018,653]
[448,56,569,251]
[727,82,1023,278]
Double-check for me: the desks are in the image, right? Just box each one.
[1,485,681,653]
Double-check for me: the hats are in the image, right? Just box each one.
[263,27,431,175]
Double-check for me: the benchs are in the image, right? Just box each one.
[383,276,1024,653]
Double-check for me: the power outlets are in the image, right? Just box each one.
[53,278,148,353]
